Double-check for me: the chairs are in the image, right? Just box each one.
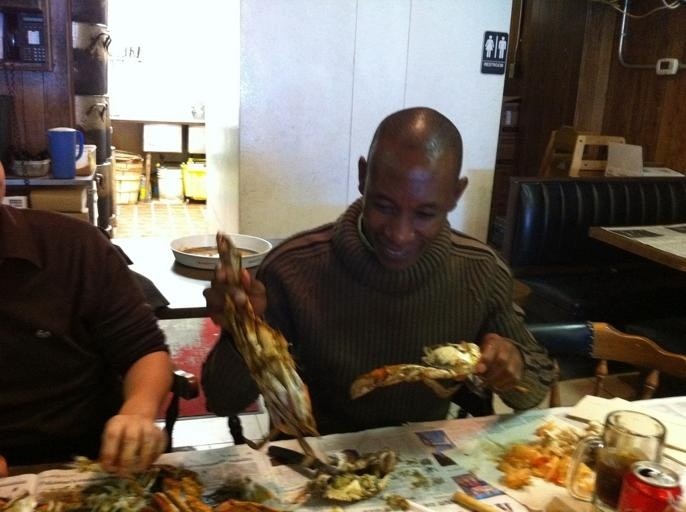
[537,125,626,176]
[526,321,686,410]
[156,364,258,452]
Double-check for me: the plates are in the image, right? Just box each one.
[169,233,273,271]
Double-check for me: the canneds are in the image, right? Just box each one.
[617,460,681,512]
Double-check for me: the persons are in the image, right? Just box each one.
[0,161,177,482]
[193,102,562,444]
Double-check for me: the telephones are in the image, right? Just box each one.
[5,8,48,63]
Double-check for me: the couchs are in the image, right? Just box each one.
[503,176,686,342]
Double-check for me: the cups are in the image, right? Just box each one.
[47,127,84,179]
[567,410,668,510]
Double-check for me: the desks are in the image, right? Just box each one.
[4,174,288,319]
[589,223,686,275]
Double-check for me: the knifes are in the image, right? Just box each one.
[269,445,343,476]
